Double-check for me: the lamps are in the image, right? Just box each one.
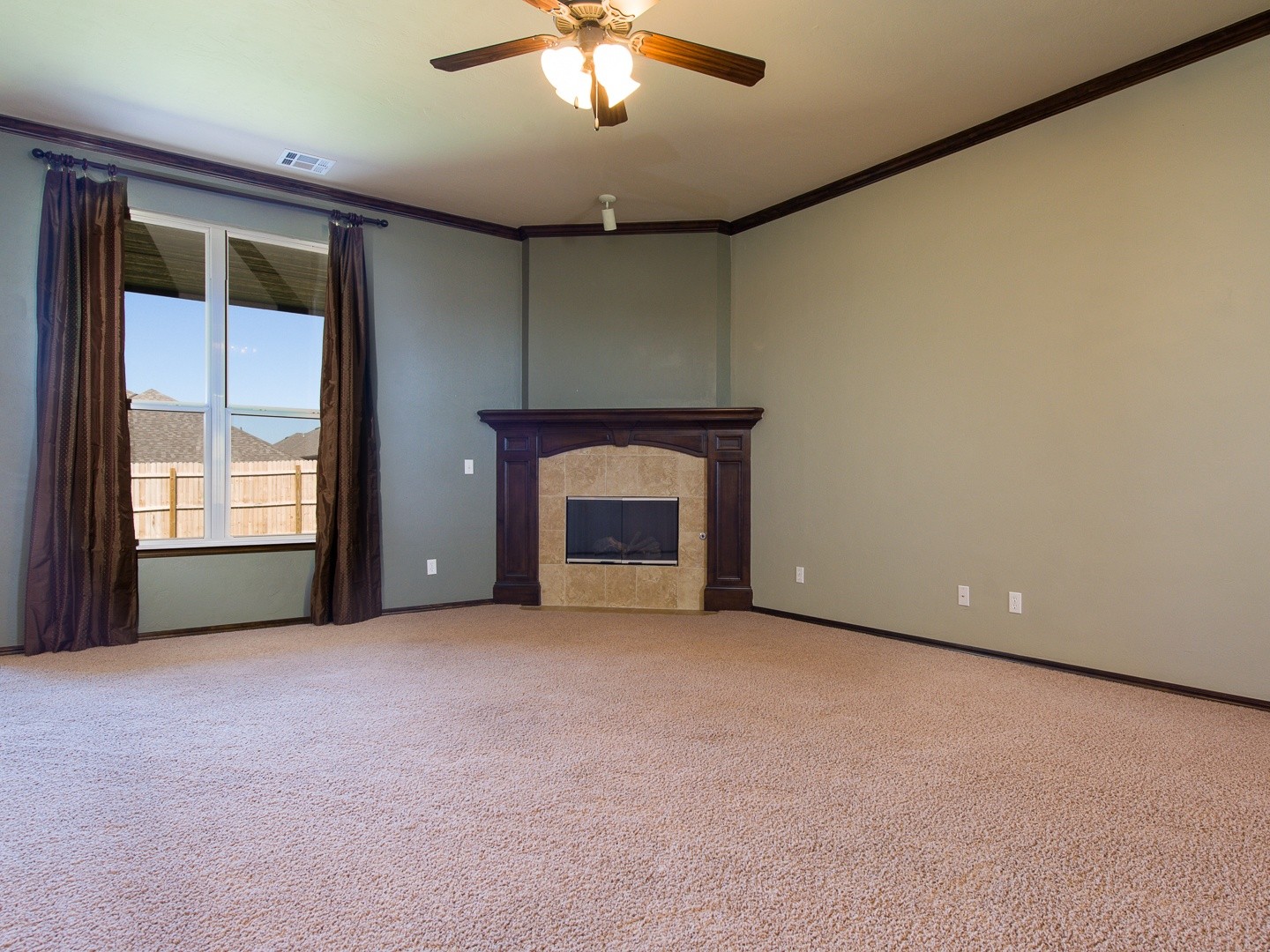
[428,0,766,132]
[598,194,617,231]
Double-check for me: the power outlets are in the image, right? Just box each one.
[796,566,804,583]
[427,558,438,575]
[958,585,970,607]
[464,459,475,475]
[1007,592,1022,614]
